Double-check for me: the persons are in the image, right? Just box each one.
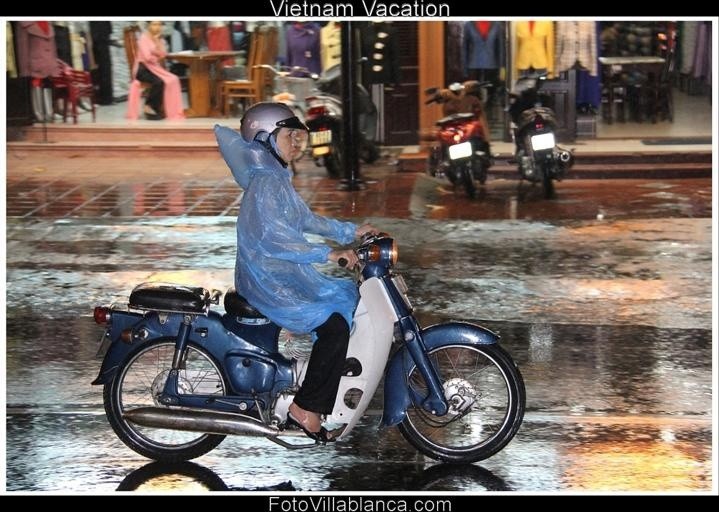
[132,21,180,120]
[234,102,379,442]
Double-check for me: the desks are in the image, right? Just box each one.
[160,49,248,118]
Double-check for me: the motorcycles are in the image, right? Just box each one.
[500,70,576,200]
[423,79,495,202]
[300,57,382,180]
[91,228,527,468]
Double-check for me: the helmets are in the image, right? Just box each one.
[240,102,309,143]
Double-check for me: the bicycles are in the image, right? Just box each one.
[248,62,321,177]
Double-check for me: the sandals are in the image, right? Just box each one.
[286,411,337,443]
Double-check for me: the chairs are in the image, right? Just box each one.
[39,57,97,124]
[123,22,180,109]
[213,25,281,116]
